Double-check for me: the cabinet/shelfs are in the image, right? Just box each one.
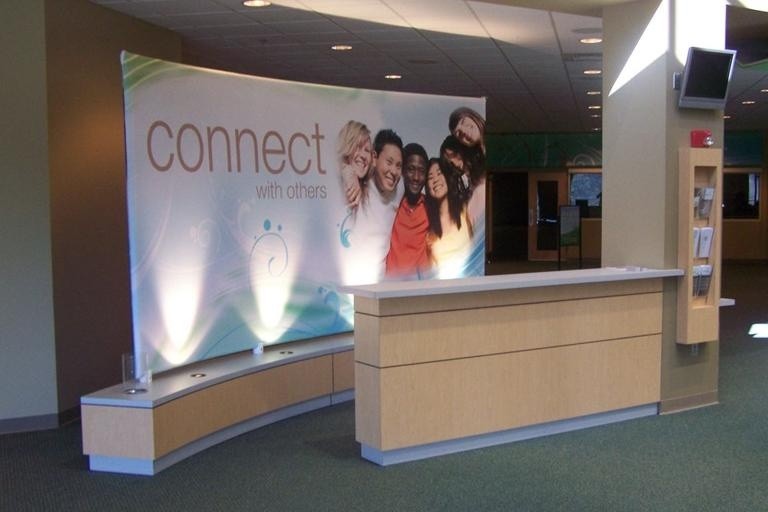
[674,147,722,345]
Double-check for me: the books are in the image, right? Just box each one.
[693,186,715,297]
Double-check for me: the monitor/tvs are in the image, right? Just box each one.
[678,46,737,109]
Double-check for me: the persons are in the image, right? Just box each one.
[439,136,486,277]
[447,107,485,154]
[423,157,472,280]
[338,120,371,208]
[385,142,432,281]
[339,130,405,281]
